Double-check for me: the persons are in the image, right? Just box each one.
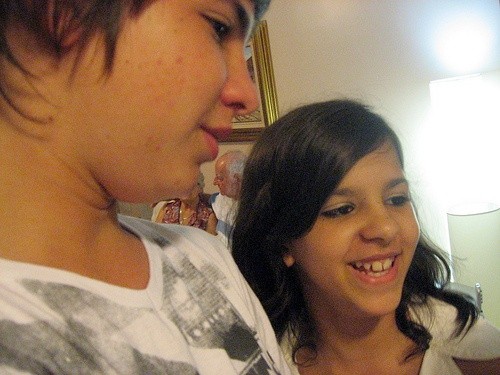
[151,150,247,252]
[0,0,290,375]
[224,97,500,375]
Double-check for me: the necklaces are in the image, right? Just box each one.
[180,206,195,225]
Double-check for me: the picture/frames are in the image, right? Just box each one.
[211,19,278,144]
